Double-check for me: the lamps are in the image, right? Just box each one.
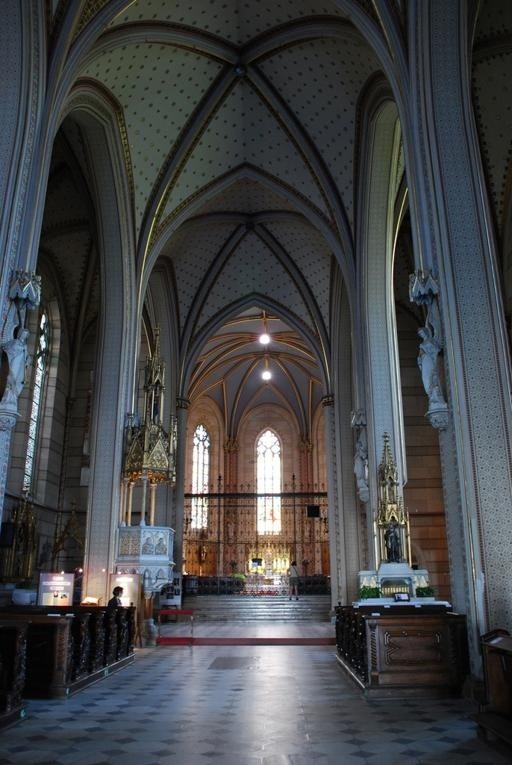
[255,306,275,385]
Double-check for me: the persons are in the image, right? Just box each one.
[286,559,300,600]
[1,328,34,412]
[14,527,28,577]
[108,586,123,605]
[352,440,368,491]
[415,326,449,410]
[383,525,401,563]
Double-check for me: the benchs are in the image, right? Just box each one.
[0,604,136,730]
[332,601,466,693]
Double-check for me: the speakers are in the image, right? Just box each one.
[307,506,319,518]
